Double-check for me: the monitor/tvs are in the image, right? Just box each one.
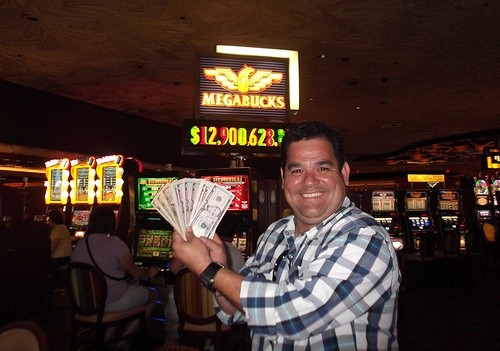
[70,207,120,230]
[134,166,253,263]
[476,193,493,220]
[372,190,465,236]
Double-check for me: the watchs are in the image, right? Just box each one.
[200,261,224,294]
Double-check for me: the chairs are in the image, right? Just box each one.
[65,262,145,351]
[172,268,241,351]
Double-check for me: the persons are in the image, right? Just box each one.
[165,217,246,351]
[70,206,161,351]
[44,210,72,296]
[171,121,401,351]
